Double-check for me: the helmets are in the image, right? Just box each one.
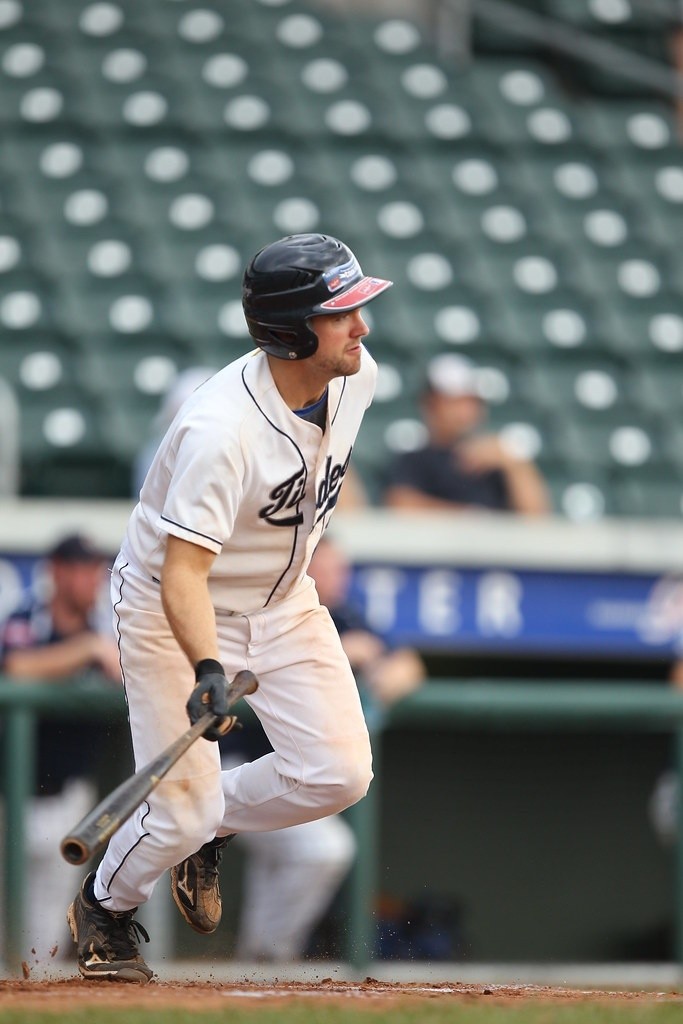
[242,233,394,360]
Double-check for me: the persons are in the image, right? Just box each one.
[219,534,424,969]
[60,232,395,989]
[378,350,554,522]
[7,531,129,983]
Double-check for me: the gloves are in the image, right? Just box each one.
[187,659,244,742]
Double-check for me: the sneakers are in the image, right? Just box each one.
[66,870,154,984]
[169,833,239,934]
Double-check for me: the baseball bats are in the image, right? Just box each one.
[61,669,259,865]
